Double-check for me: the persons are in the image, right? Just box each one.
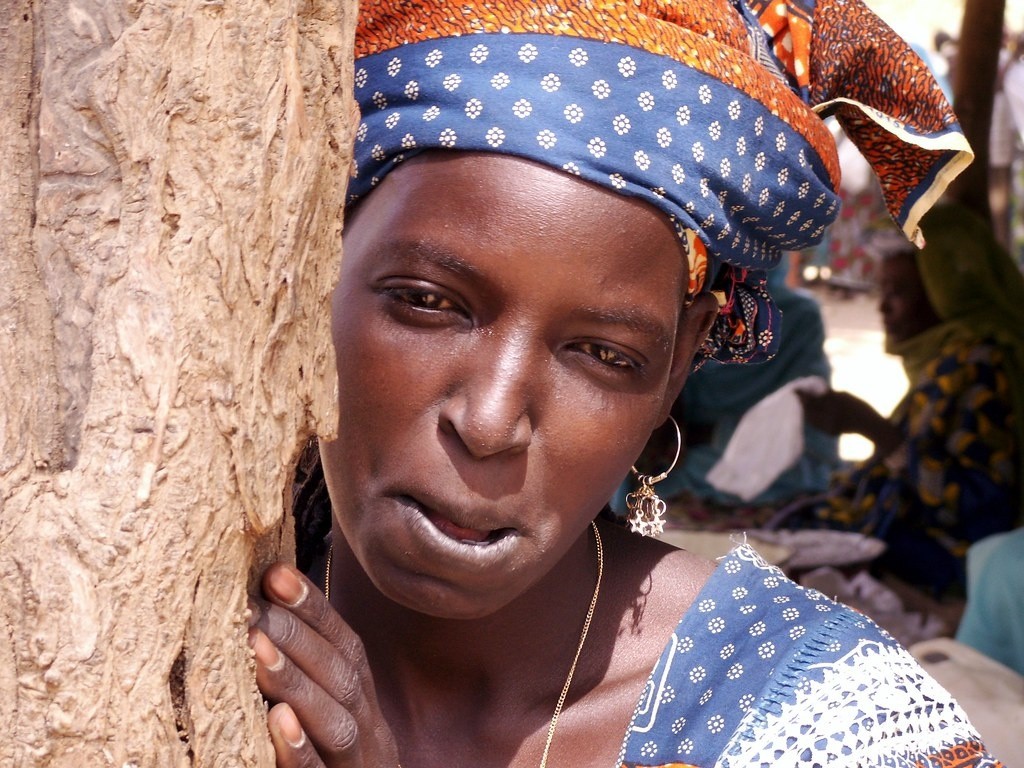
[249,1,1023,768]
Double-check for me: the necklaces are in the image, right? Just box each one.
[323,520,604,768]
